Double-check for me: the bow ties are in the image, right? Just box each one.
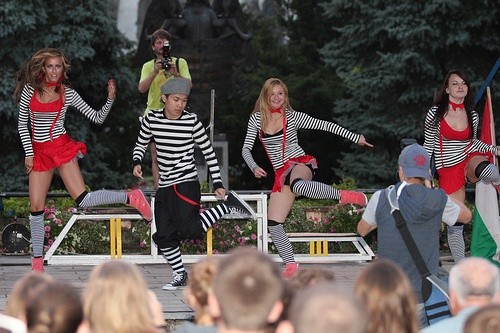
[45,80,60,88]
[448,100,465,111]
[269,107,282,113]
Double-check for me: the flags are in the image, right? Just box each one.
[469,86,500,268]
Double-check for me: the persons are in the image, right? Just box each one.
[424,68,500,265]
[243,78,374,279]
[357,141,473,330]
[0,246,500,333]
[139,28,193,190]
[12,46,153,273]
[133,77,258,291]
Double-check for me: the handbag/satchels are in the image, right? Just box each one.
[422,268,452,325]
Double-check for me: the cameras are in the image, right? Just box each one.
[400,138,417,152]
[158,40,171,70]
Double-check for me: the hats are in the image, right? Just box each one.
[397,143,431,180]
[160,77,191,96]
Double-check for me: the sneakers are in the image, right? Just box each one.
[162,272,189,291]
[340,189,368,207]
[128,189,152,221]
[223,190,257,221]
[282,262,299,278]
[32,257,44,273]
[492,181,500,195]
